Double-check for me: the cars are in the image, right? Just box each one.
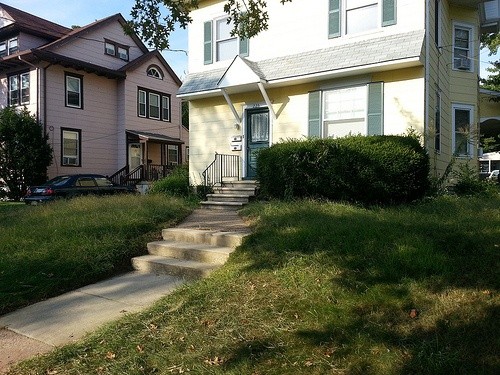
[24,173,137,205]
